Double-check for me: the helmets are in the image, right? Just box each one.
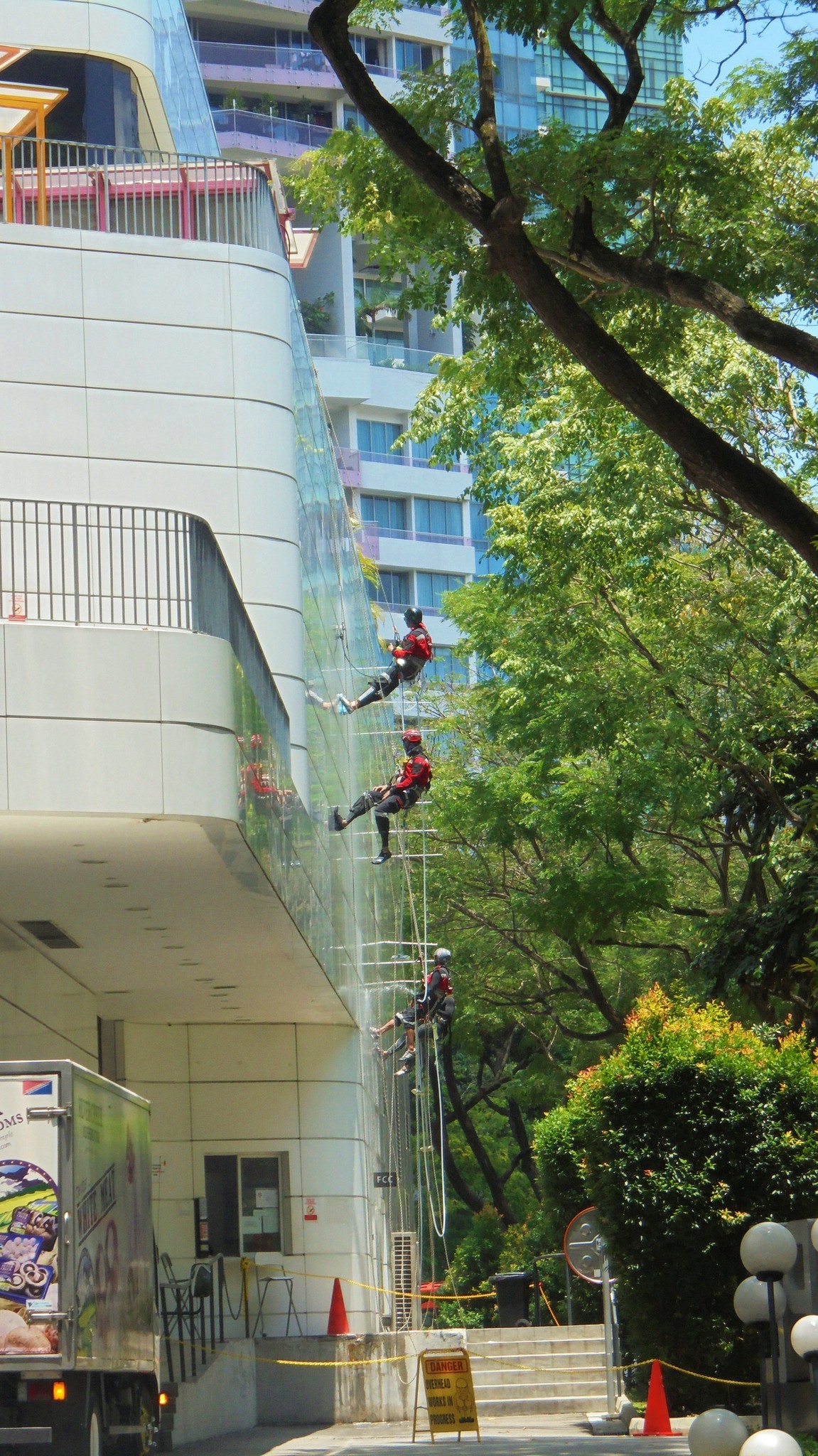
[434,948,451,964]
[401,729,421,743]
[405,608,423,624]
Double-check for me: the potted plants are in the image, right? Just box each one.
[252,91,279,139]
[219,87,248,131]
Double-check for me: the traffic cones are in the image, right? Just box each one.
[325,1277,350,1334]
[633,1360,688,1437]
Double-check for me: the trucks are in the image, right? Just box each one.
[1,1058,166,1456]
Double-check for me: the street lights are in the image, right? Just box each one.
[739,1222,798,1431]
[733,1275,786,1430]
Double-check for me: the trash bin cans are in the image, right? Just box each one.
[488,1272,533,1328]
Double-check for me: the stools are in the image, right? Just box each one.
[161,1252,200,1339]
[254,1252,303,1338]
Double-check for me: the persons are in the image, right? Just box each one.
[376,982,454,1076]
[336,729,433,864]
[340,608,433,713]
[367,948,451,1061]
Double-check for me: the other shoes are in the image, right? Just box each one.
[340,694,354,715]
[366,1021,380,1040]
[395,1066,413,1077]
[334,806,346,831]
[372,850,392,865]
[397,1048,417,1062]
[376,1045,387,1061]
